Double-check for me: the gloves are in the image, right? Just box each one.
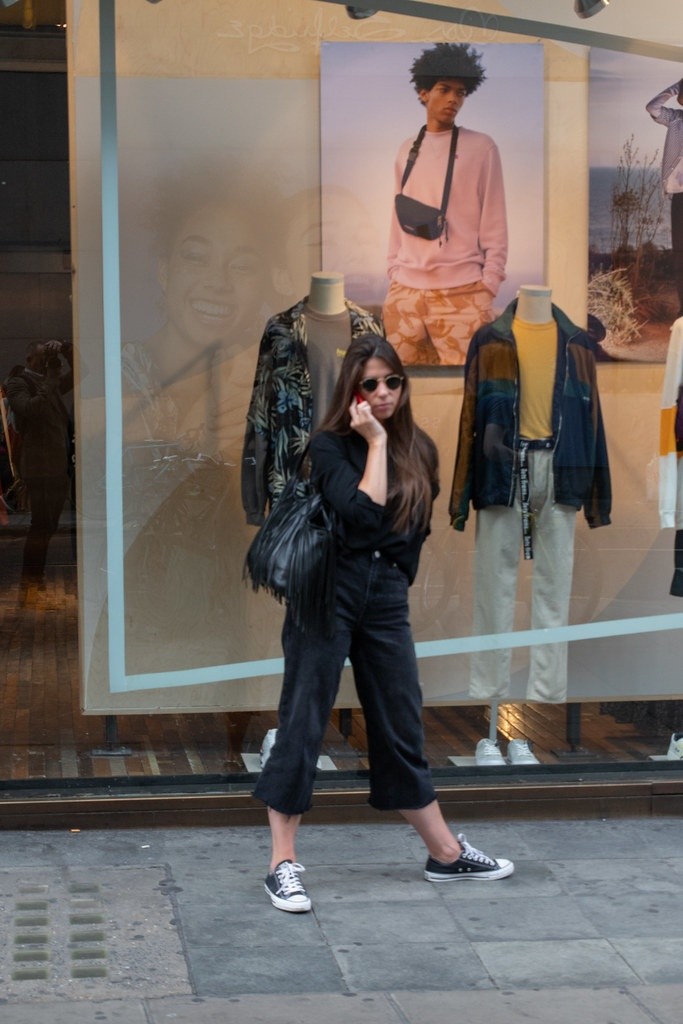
[45,339,62,353]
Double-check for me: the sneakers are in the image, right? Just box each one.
[264,859,311,912]
[668,734,683,760]
[260,728,277,768]
[424,833,514,882]
[506,739,539,765]
[474,739,505,765]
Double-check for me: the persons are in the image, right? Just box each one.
[3,340,88,616]
[240,270,385,528]
[646,78,683,318]
[250,333,515,912]
[448,283,611,705]
[381,43,508,365]
[110,178,393,670]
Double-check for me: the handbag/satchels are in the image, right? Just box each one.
[394,194,445,240]
[243,441,331,606]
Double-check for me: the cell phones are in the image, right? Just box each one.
[353,390,363,405]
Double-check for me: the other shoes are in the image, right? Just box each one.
[21,576,47,593]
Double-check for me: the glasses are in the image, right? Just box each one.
[359,373,404,393]
